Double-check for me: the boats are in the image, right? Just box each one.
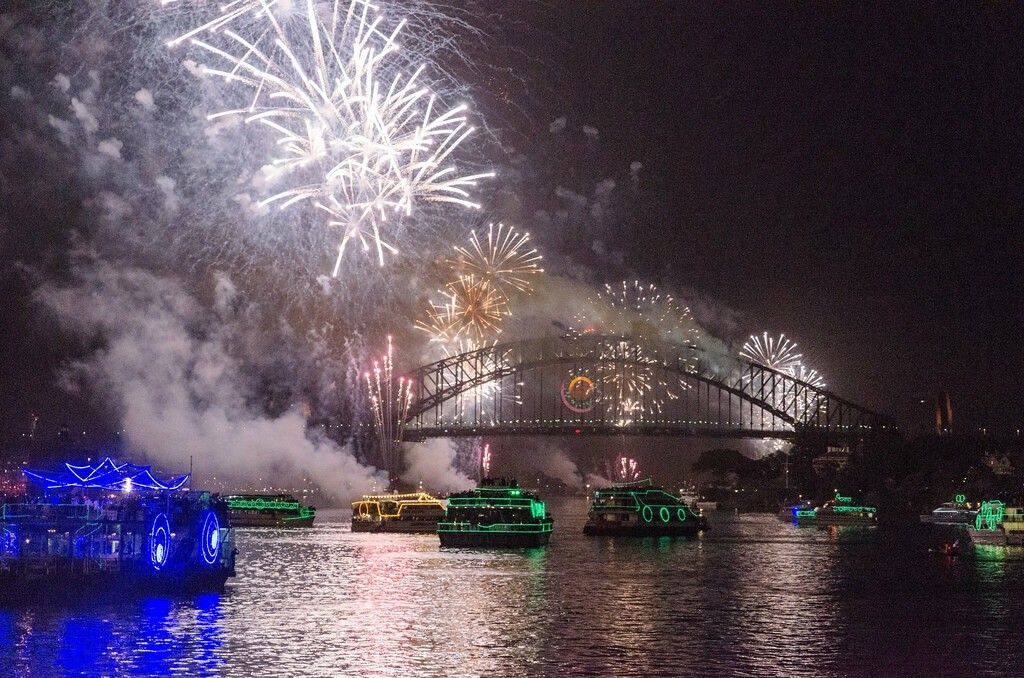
[352,491,445,533]
[221,492,317,528]
[793,493,879,527]
[583,475,711,536]
[777,495,817,522]
[0,459,237,599]
[920,495,1024,547]
[678,476,722,510]
[435,476,555,548]
